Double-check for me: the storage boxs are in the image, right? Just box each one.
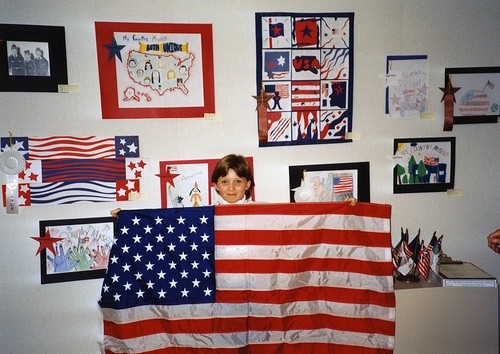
[437,262,496,288]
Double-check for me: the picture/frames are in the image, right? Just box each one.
[39,215,116,284]
[0,22,67,93]
[288,162,371,204]
[393,137,457,194]
[445,66,500,125]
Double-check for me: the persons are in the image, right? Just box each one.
[9,44,48,76]
[110,154,357,218]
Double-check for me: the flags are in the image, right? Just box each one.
[99,201,396,354]
[391,231,442,281]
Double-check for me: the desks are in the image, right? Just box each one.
[393,274,499,354]
